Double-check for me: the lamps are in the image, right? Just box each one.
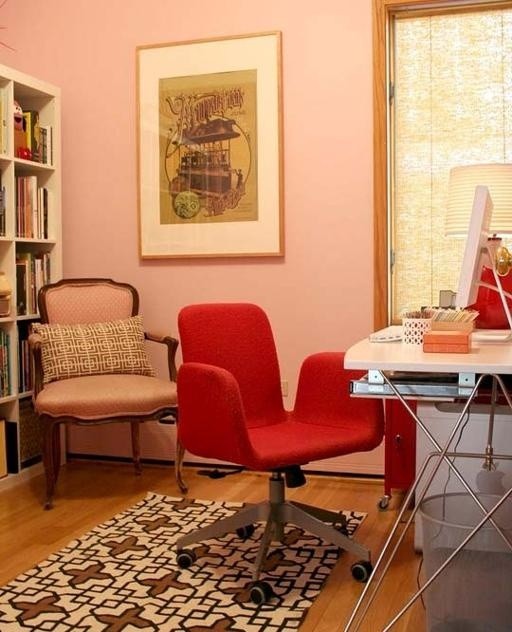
[445,164,512,265]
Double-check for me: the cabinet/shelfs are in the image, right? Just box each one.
[376,393,510,508]
[1,64,66,495]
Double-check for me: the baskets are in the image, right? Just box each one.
[401,318,430,346]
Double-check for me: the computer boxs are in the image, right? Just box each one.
[415,403,512,553]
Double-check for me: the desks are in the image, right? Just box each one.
[344,314,512,631]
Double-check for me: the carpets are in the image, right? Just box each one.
[0,488,367,632]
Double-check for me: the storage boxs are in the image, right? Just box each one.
[421,330,470,354]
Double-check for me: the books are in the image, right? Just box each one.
[0,102,53,479]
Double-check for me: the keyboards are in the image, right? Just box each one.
[368,320,401,344]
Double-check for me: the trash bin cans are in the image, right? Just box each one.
[417,492,512,631]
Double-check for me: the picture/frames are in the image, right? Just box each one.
[136,30,285,261]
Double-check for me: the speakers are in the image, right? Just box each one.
[440,290,455,307]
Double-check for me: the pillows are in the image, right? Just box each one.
[34,316,158,383]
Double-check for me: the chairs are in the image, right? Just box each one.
[174,301,383,607]
[30,277,186,510]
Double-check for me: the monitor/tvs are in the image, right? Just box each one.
[455,184,512,341]
